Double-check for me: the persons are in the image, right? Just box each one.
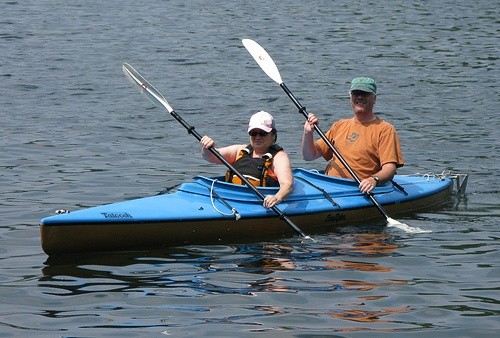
[302,77,404,195]
[200,111,294,208]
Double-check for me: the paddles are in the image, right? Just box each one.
[242,39,431,234]
[121,63,316,244]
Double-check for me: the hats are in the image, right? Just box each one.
[350,77,377,95]
[247,111,275,133]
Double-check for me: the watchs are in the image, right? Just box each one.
[370,175,379,185]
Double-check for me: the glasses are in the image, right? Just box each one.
[249,131,270,136]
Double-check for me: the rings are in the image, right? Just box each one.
[368,184,371,188]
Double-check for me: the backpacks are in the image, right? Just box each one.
[226,144,283,189]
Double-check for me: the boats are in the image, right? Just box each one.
[38,167,470,258]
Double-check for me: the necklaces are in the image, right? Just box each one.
[254,148,267,154]
